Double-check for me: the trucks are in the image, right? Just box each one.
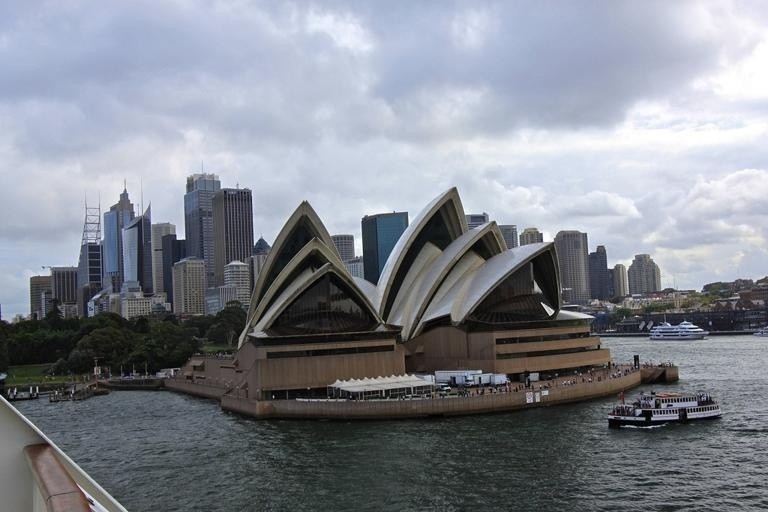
[436,373,511,392]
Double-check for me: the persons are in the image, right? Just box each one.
[191,351,225,360]
[401,362,640,399]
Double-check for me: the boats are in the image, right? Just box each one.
[650,321,709,340]
[607,390,721,427]
